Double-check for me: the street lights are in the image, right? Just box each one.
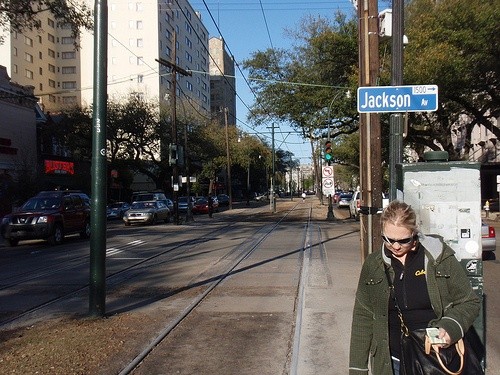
[327,89,351,221]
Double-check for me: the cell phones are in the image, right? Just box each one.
[426,328,448,345]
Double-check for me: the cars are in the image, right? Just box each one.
[482,218,496,254]
[107,189,230,227]
[255,191,279,202]
[331,189,386,222]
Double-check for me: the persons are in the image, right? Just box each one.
[302,191,306,203]
[173,200,178,224]
[348,199,486,374]
[208,193,213,218]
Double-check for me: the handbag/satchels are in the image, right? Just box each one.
[399,328,485,375]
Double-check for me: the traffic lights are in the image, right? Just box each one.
[324,140,333,161]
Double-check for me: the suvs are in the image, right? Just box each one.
[0,189,92,247]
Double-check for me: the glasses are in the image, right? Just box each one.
[381,232,414,244]
